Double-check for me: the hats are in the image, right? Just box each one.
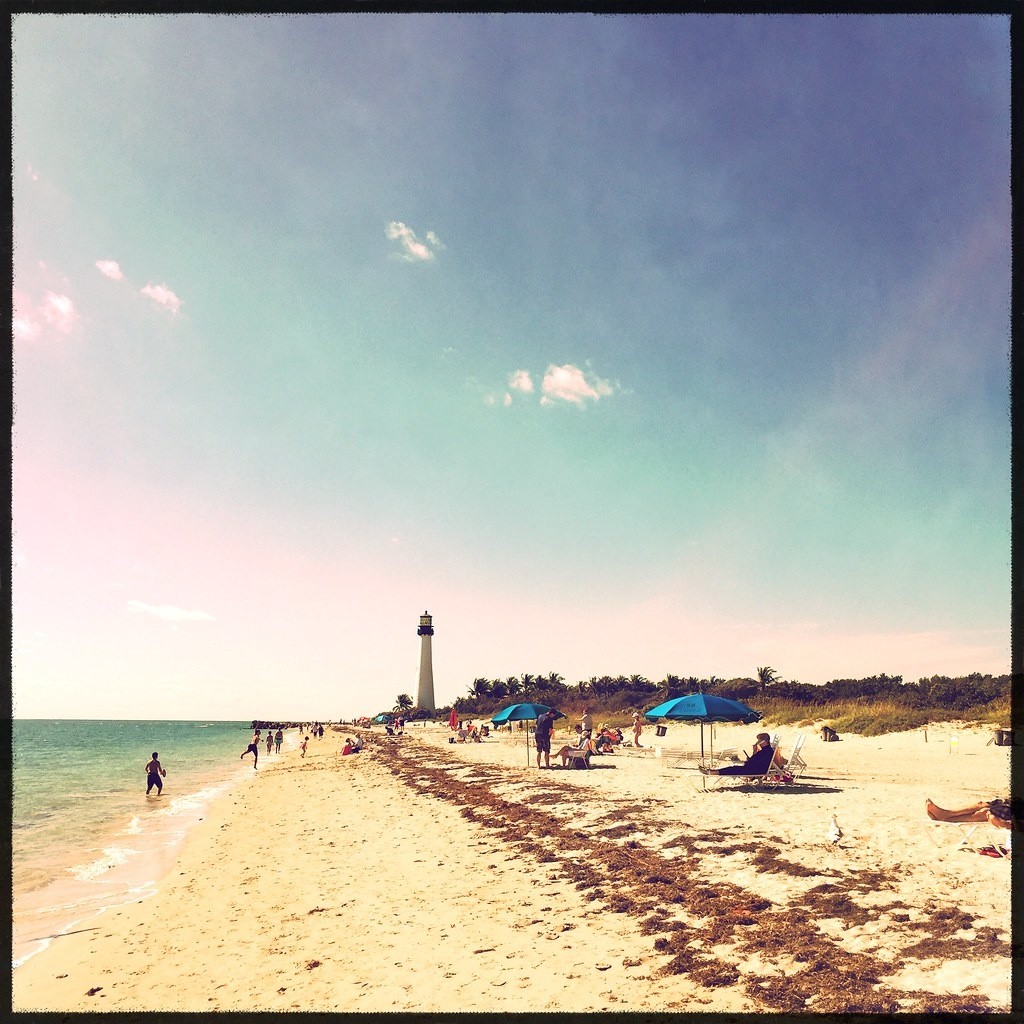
[632,712,639,718]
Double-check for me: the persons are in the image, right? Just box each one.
[458,725,481,743]
[632,713,641,744]
[300,736,309,757]
[241,724,260,770]
[548,709,623,768]
[298,721,324,740]
[535,709,556,769]
[924,797,1011,822]
[698,734,774,774]
[145,752,166,794]
[340,733,362,755]
[274,728,283,753]
[395,715,405,732]
[265,731,273,753]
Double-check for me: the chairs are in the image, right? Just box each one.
[567,751,589,769]
[687,732,808,792]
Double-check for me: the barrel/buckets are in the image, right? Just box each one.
[449,737,454,743]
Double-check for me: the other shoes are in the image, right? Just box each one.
[980,845,1007,858]
[699,766,710,778]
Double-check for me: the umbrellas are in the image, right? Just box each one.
[375,715,391,724]
[643,694,764,788]
[491,703,567,767]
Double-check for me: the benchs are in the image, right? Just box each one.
[923,819,1011,858]
